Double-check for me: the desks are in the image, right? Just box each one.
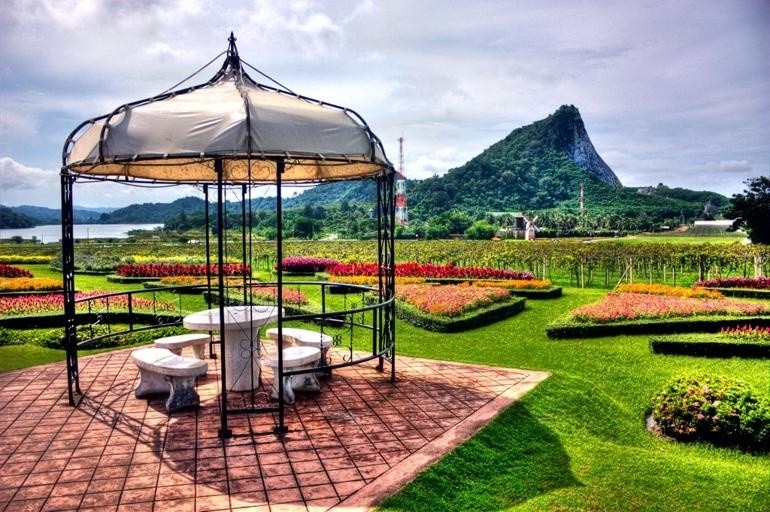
[183,304,286,391]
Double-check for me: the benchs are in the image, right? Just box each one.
[266,346,322,405]
[266,327,333,376]
[154,333,212,377]
[130,347,208,411]
